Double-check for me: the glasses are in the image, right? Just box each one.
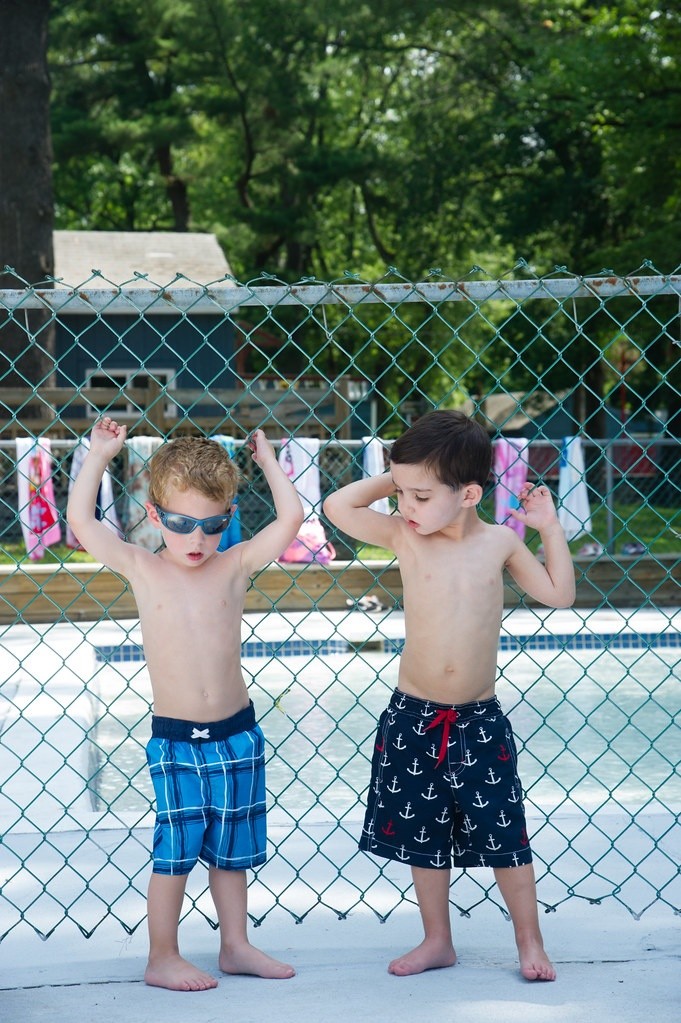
[154,503,232,536]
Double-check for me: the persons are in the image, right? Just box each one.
[67,416,307,990]
[323,412,576,982]
